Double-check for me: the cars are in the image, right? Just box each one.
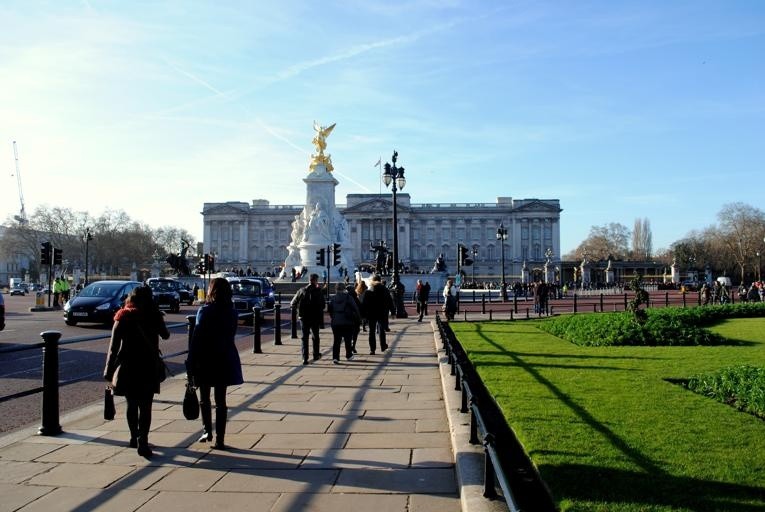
[7,282,57,297]
[145,275,277,330]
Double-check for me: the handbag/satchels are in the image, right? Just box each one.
[104,388,116,420]
[183,389,199,419]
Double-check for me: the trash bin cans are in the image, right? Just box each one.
[197,289,205,301]
[35,292,45,308]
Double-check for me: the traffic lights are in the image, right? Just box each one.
[52,248,62,267]
[315,246,325,266]
[332,243,341,266]
[460,247,470,266]
[37,240,50,263]
[194,251,216,274]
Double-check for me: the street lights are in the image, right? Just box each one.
[544,245,553,263]
[82,231,92,289]
[270,260,274,276]
[496,222,511,302]
[754,237,765,259]
[469,246,478,285]
[580,245,590,263]
[383,148,407,319]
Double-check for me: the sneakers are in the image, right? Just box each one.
[418,319,422,322]
[129,437,137,448]
[315,353,321,359]
[347,356,354,361]
[382,344,388,351]
[303,360,308,364]
[199,432,212,442]
[138,441,152,456]
[363,330,366,332]
[384,329,390,332]
[332,359,338,364]
[352,347,357,353]
[210,442,225,450]
[370,352,375,354]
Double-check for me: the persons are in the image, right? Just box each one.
[103,287,169,457]
[181,242,189,258]
[61,275,70,304]
[184,278,244,449]
[226,267,278,278]
[369,240,387,274]
[700,280,765,306]
[182,281,199,300]
[289,274,567,364]
[53,278,62,307]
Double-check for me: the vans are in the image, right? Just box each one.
[61,279,146,326]
